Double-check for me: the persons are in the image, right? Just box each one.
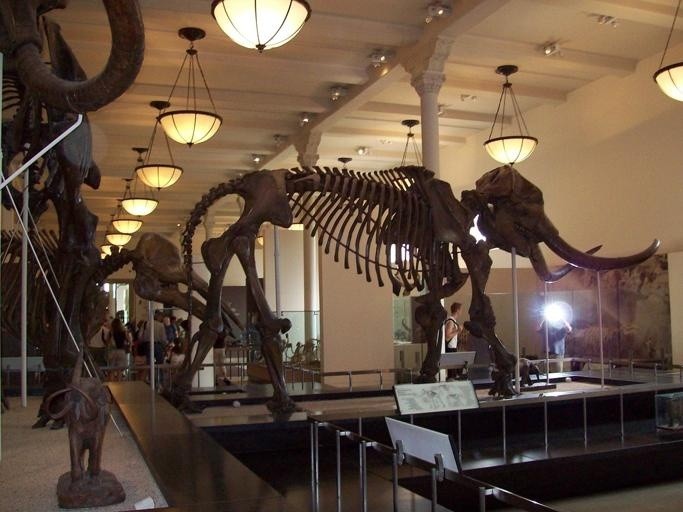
[445,302,467,378]
[86,308,190,390]
[536,305,573,373]
[215,329,235,386]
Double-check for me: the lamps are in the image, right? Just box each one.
[209,0,312,52]
[100,197,133,260]
[110,177,143,235]
[392,119,423,191]
[136,100,184,190]
[483,65,538,164]
[119,147,158,217]
[652,0,683,102]
[155,26,222,147]
